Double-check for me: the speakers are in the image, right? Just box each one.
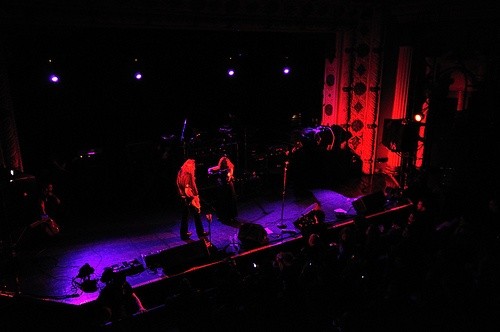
[164,239,213,273]
[237,222,268,248]
[351,189,388,215]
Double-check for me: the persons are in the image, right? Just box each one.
[207,155,236,226]
[157,196,500,332]
[175,157,208,240]
[98,271,149,324]
[35,182,61,233]
